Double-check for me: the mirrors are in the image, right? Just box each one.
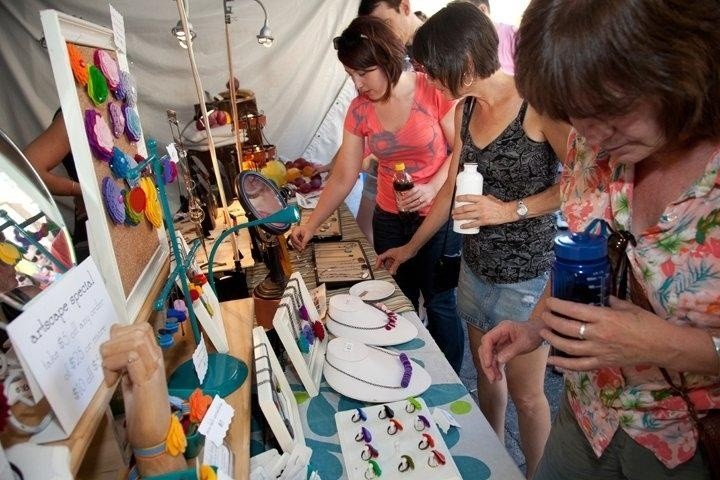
[236,170,292,299]
[0,128,77,310]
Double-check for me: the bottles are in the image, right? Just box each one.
[393,160,420,225]
[548,217,613,369]
[451,162,485,235]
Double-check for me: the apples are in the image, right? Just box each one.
[196,110,230,129]
[285,157,322,193]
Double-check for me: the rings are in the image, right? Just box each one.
[351,396,446,480]
[578,322,585,340]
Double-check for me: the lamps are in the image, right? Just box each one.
[224,0,274,48]
[172,0,196,48]
[208,205,300,297]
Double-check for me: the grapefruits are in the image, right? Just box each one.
[262,160,288,188]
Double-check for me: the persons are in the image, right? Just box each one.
[466,0,519,76]
[372,0,573,480]
[289,13,464,376]
[22,106,90,264]
[100,321,189,476]
[310,0,429,246]
[477,1,720,480]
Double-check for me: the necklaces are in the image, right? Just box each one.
[325,301,398,330]
[275,277,325,354]
[168,235,215,318]
[323,343,413,389]
[311,236,370,281]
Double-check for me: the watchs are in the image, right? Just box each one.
[516,198,529,220]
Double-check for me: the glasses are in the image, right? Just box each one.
[605,225,636,305]
[332,32,370,50]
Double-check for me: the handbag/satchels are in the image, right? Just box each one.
[433,257,461,291]
[689,405,719,479]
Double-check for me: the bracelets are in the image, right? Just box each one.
[131,441,169,458]
[705,329,720,360]
[71,180,75,197]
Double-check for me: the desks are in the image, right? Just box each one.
[0,201,527,480]
